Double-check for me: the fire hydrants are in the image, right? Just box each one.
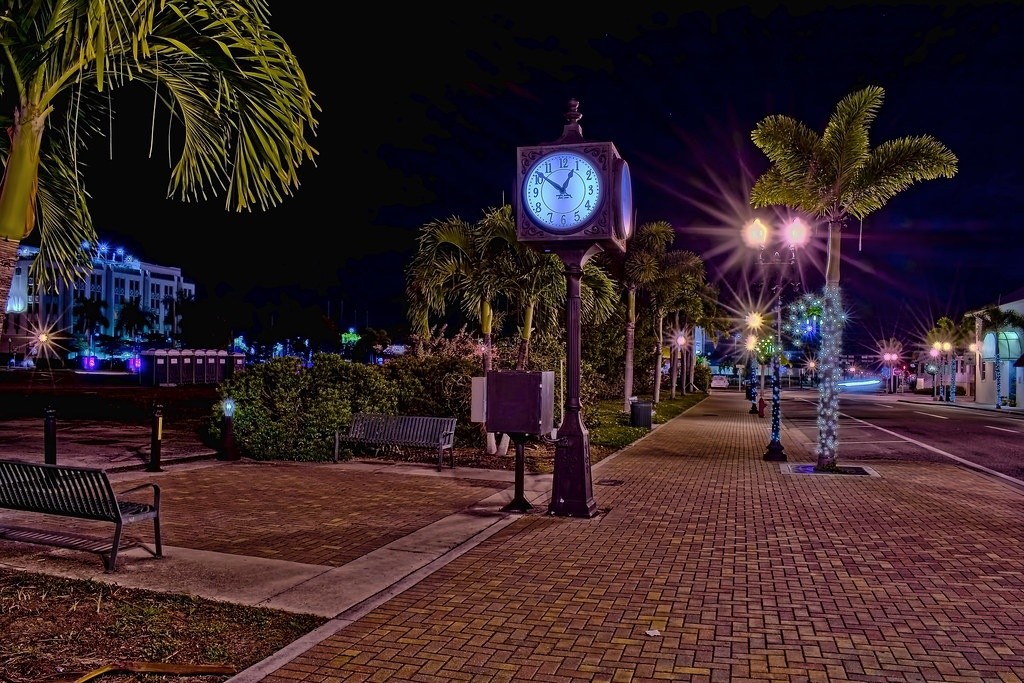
[758,397,768,418]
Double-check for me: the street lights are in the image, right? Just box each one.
[677,336,686,387]
[744,311,764,414]
[883,352,899,394]
[38,333,48,359]
[969,343,979,402]
[744,215,812,462]
[933,341,950,401]
[808,361,815,387]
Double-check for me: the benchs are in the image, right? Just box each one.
[333,412,457,472]
[0,458,164,574]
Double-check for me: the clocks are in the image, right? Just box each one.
[615,158,633,239]
[521,151,607,236]
